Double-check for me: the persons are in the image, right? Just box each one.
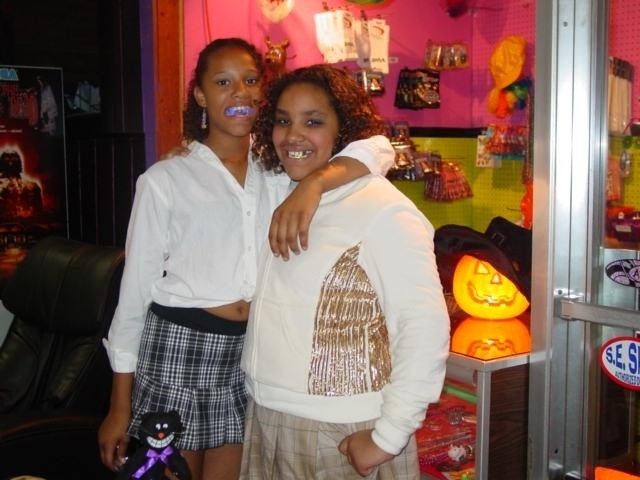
[237,64,451,479]
[97,37,395,480]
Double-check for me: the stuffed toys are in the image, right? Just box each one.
[114,407,191,479]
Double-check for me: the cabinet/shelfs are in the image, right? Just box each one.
[418,292,531,478]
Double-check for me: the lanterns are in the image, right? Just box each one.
[453,239,530,319]
[449,317,532,359]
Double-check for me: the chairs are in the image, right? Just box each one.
[1,231,127,479]
